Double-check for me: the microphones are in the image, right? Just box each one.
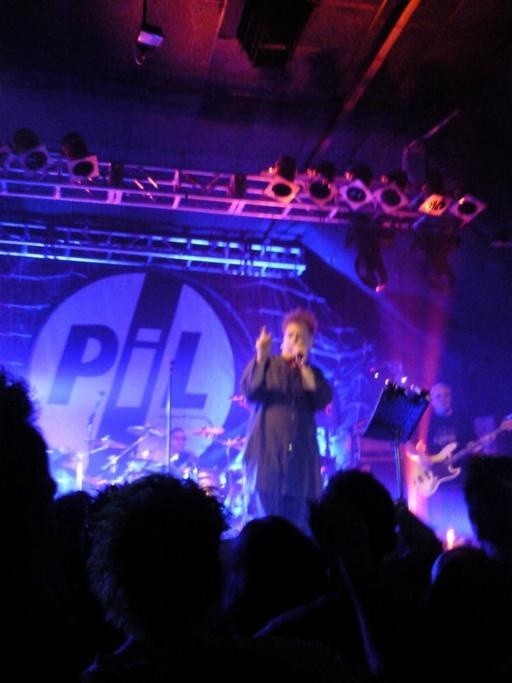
[168,360,174,380]
[88,390,106,423]
[289,345,302,360]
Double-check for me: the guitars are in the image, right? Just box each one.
[412,417,511,497]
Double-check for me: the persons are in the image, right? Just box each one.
[237,305,330,531]
[407,383,482,536]
[58,452,512,682]
[165,426,195,462]
[0,363,73,682]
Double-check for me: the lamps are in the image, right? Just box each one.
[127,0,167,67]
[342,222,396,293]
[411,223,461,301]
[0,125,488,239]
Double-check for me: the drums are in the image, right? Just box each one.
[184,468,220,496]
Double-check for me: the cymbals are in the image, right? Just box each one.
[191,427,224,435]
[84,439,126,448]
[127,426,163,437]
